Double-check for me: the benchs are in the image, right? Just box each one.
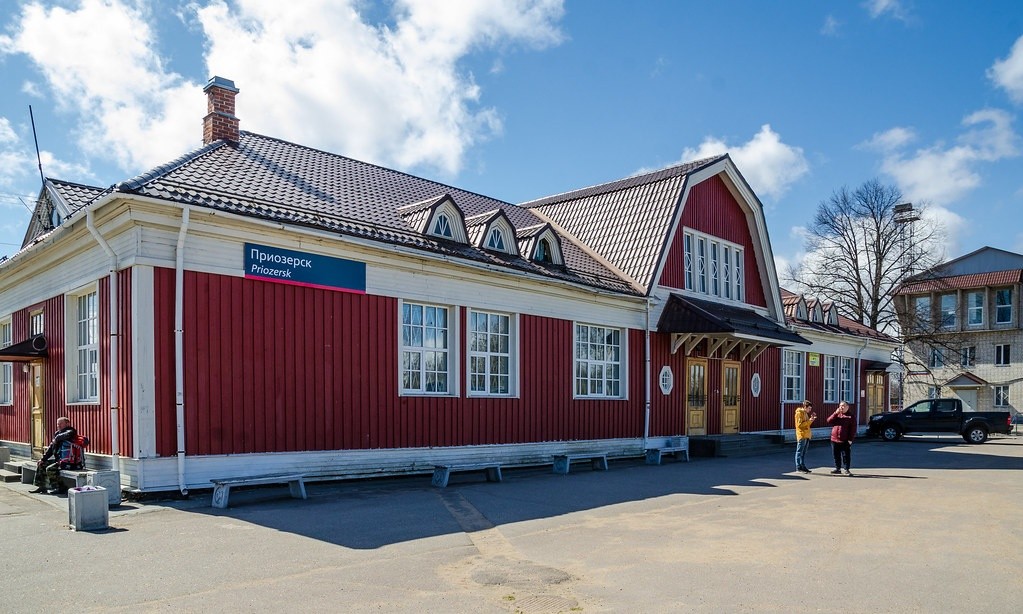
[22,461,109,487]
[644,446,689,465]
[431,464,502,487]
[210,473,306,508]
[551,452,608,474]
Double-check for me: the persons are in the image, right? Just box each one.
[28,417,78,494]
[827,401,857,476]
[795,400,817,474]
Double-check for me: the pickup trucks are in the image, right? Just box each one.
[868,398,1014,444]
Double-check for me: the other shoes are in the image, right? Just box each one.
[843,469,852,476]
[29,486,47,493]
[800,466,812,473]
[830,469,842,474]
[50,485,69,494]
[796,470,807,474]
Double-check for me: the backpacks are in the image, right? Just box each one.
[58,427,88,471]
